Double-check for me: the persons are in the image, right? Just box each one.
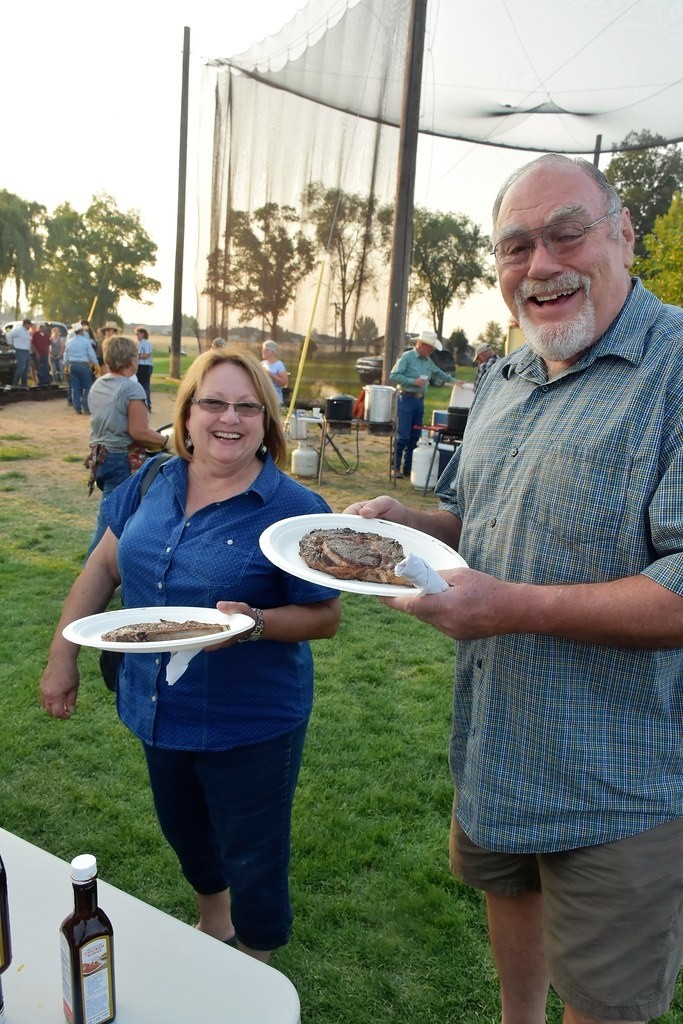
[343,157,682,1023]
[260,343,288,405]
[389,332,465,478]
[474,343,500,393]
[41,349,343,962]
[136,328,153,411]
[84,336,177,500]
[0,319,100,416]
[98,322,122,337]
[212,339,225,350]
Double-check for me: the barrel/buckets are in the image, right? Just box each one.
[430,410,449,435]
[362,385,396,422]
[290,415,307,439]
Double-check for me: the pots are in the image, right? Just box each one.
[324,393,354,421]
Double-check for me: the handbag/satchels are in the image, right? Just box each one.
[100,650,122,692]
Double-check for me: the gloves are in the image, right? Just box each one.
[93,364,100,375]
[64,366,69,373]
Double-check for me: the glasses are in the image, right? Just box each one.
[190,397,265,417]
[491,207,619,272]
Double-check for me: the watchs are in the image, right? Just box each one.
[238,609,264,643]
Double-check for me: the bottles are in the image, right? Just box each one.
[59,854,116,1024]
[285,423,291,436]
[299,412,306,418]
[421,425,429,438]
[0,856,13,1024]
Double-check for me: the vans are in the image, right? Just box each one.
[2,321,69,346]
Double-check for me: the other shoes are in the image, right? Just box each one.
[391,469,403,478]
[402,468,411,477]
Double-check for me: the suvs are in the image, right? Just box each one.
[354,348,456,387]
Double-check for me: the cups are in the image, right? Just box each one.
[313,408,320,418]
[420,375,428,385]
[160,429,174,436]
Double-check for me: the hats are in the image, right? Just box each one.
[415,331,443,352]
[72,322,83,332]
[23,319,33,325]
[473,342,491,363]
[100,321,122,333]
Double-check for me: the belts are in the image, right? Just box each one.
[399,390,423,398]
[71,362,89,365]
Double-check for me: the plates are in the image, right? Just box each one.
[259,513,470,597]
[62,607,256,653]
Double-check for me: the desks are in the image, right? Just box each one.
[0,828,301,1024]
[421,426,464,499]
[298,416,396,489]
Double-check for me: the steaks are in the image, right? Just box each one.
[101,619,231,643]
[299,527,415,587]
